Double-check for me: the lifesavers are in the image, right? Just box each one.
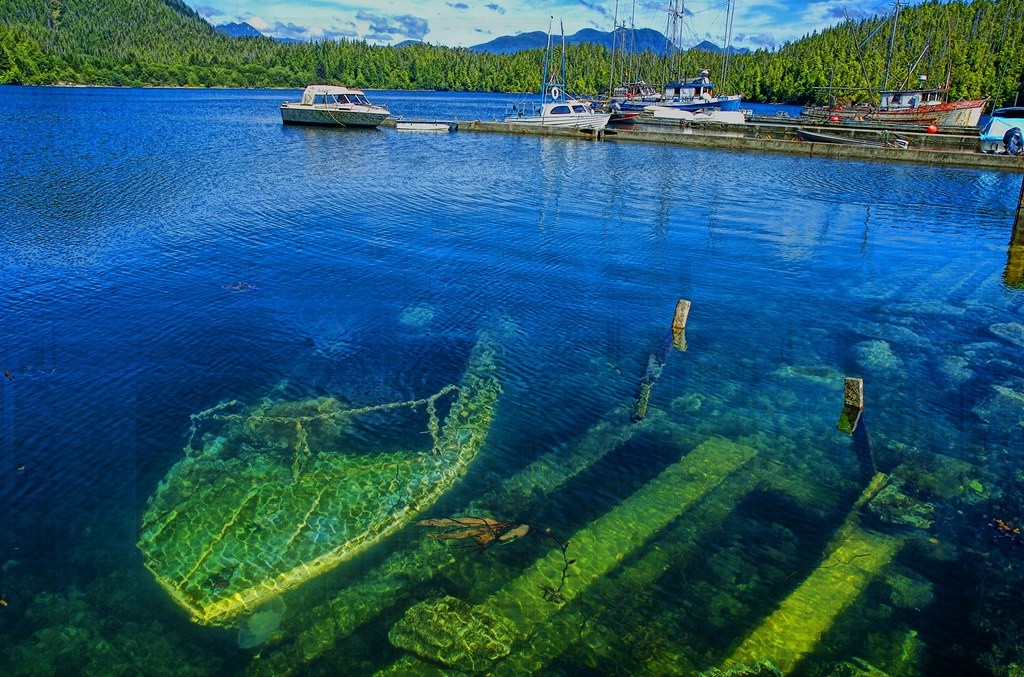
[551,86,559,100]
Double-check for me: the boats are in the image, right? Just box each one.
[279,85,391,127]
[976,106,1024,156]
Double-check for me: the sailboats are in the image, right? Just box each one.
[796,0,993,152]
[503,0,754,134]
